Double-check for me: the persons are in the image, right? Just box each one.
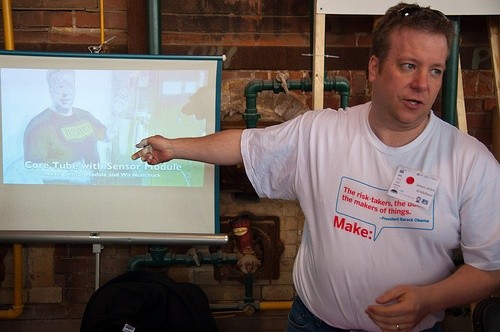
[22,69,114,185]
[131,2,500,332]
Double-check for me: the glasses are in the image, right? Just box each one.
[397,6,450,23]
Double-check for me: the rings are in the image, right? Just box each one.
[397,324,402,332]
